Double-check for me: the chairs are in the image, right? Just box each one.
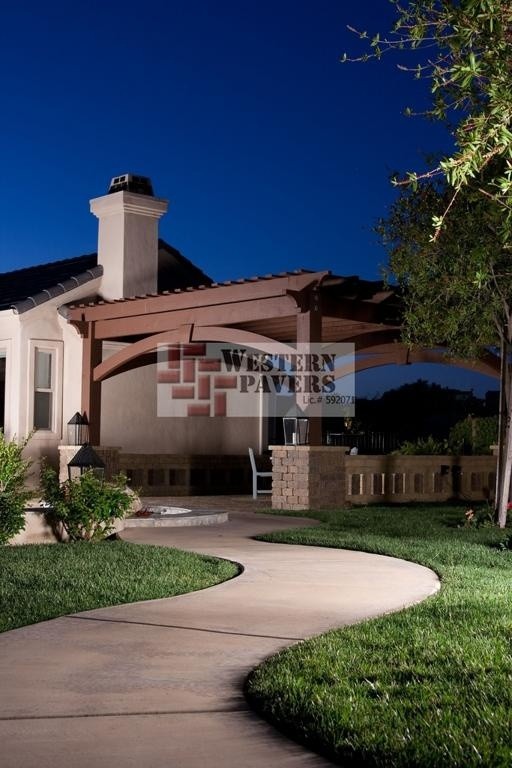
[247,446,272,500]
